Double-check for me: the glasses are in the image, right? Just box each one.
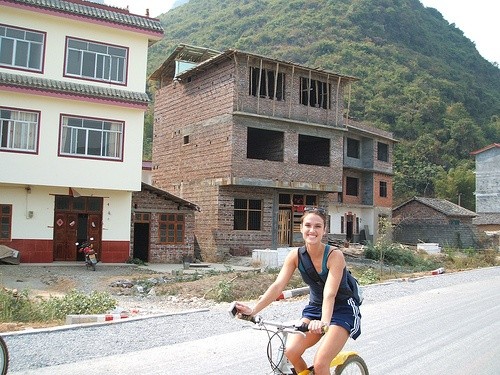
[304,207,325,215]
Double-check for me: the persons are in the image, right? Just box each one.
[235,206,360,375]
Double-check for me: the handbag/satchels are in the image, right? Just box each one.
[346,270,364,306]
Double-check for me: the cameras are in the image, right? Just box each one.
[227,301,241,318]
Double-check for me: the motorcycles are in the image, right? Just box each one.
[75,238,98,272]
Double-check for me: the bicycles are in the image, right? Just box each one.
[237,313,369,375]
[0,336,9,375]
